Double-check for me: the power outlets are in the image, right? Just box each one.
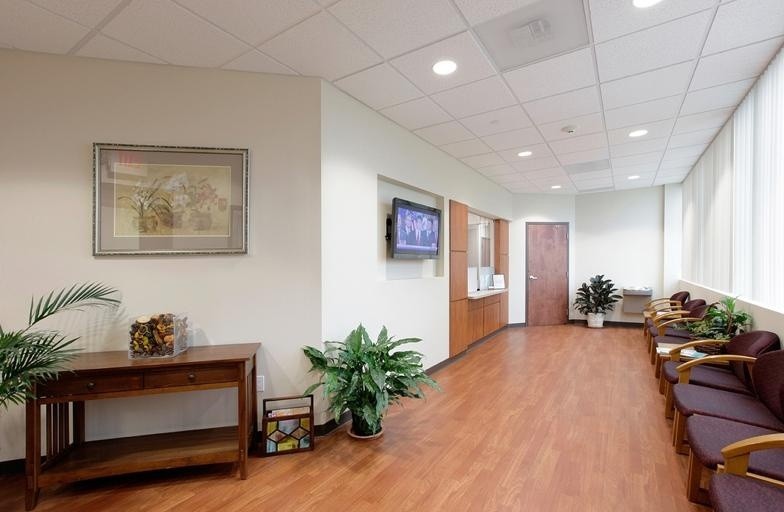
[257,376,265,391]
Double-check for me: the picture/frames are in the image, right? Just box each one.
[91,141,249,255]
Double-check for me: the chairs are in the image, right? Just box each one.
[646,298,706,352]
[711,433,784,512]
[685,413,783,500]
[649,304,717,361]
[643,292,690,335]
[658,331,779,418]
[672,350,784,453]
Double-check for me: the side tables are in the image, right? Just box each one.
[656,341,697,357]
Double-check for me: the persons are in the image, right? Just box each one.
[396,212,436,247]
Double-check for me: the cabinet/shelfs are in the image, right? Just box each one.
[447,199,511,358]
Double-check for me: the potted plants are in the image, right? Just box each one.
[301,323,448,440]
[673,295,753,355]
[573,274,624,328]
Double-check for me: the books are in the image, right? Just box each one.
[656,347,697,358]
[266,405,310,454]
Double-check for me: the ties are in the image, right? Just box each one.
[427,231,430,241]
[417,231,420,245]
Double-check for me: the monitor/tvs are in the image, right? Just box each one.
[391,196,442,260]
